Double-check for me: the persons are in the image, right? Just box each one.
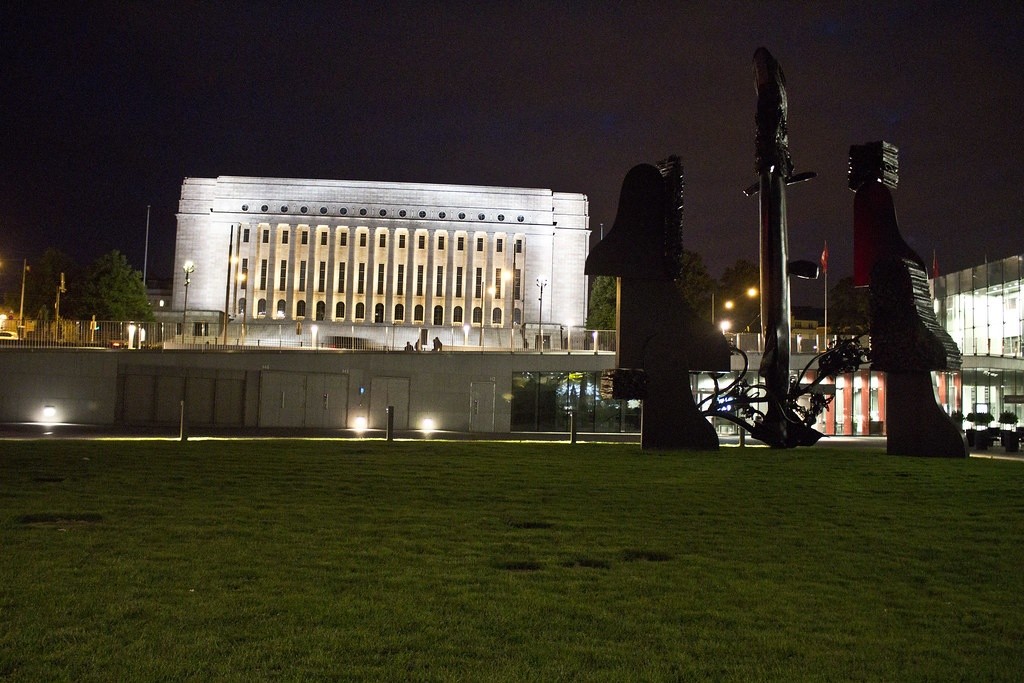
[432,337,442,352]
[405,342,414,351]
[415,338,422,351]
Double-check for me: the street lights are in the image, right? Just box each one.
[181,260,194,346]
[537,275,548,354]
